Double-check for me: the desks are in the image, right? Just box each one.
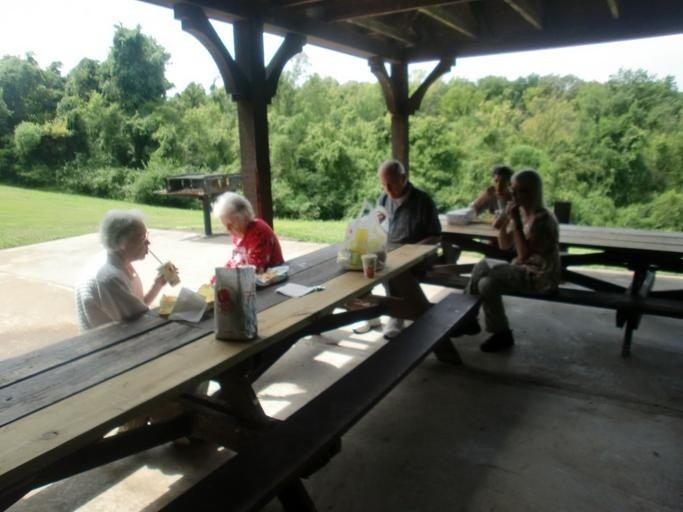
[0,241,440,512]
[435,213,682,356]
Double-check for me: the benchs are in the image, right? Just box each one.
[420,269,682,361]
[157,292,484,511]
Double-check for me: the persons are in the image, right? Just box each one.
[207,192,286,285]
[470,167,513,220]
[73,208,184,435]
[451,169,560,352]
[353,160,440,339]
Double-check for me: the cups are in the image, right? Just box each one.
[158,261,180,286]
[360,254,378,278]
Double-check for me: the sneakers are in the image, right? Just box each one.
[479,329,515,354]
[382,315,406,341]
[446,317,482,338]
[350,316,383,334]
